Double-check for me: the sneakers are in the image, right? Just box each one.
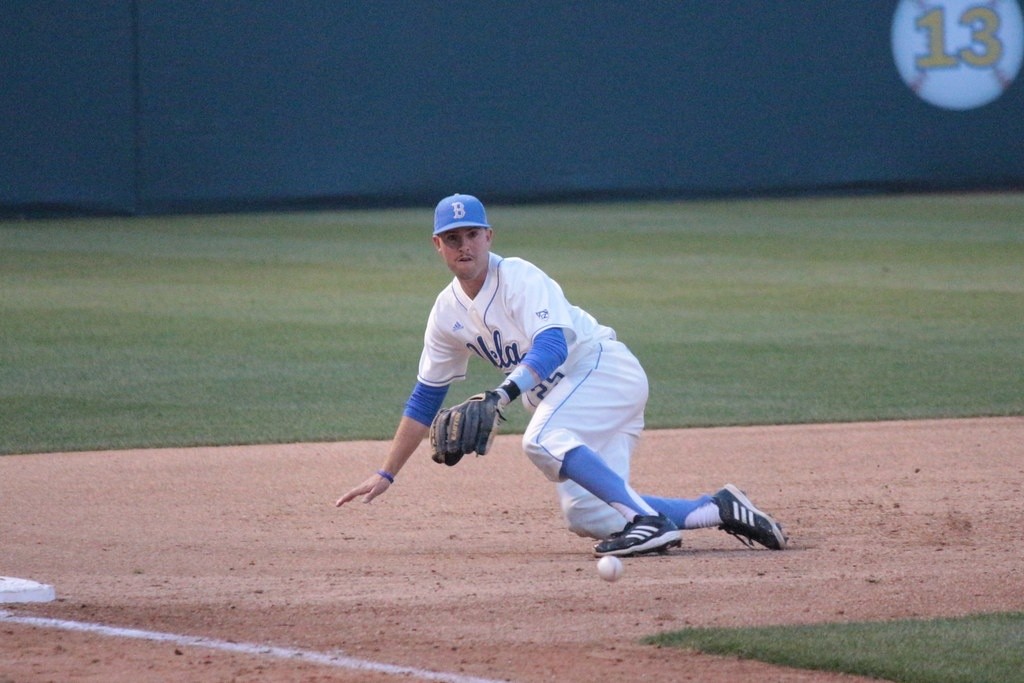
[592,512,683,557]
[712,483,786,551]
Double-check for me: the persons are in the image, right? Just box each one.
[335,193,786,558]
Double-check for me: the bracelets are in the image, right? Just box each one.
[377,470,394,484]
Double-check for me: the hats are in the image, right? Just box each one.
[430,193,491,235]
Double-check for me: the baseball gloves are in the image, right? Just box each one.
[431,392,507,466]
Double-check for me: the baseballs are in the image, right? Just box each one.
[598,555,622,582]
